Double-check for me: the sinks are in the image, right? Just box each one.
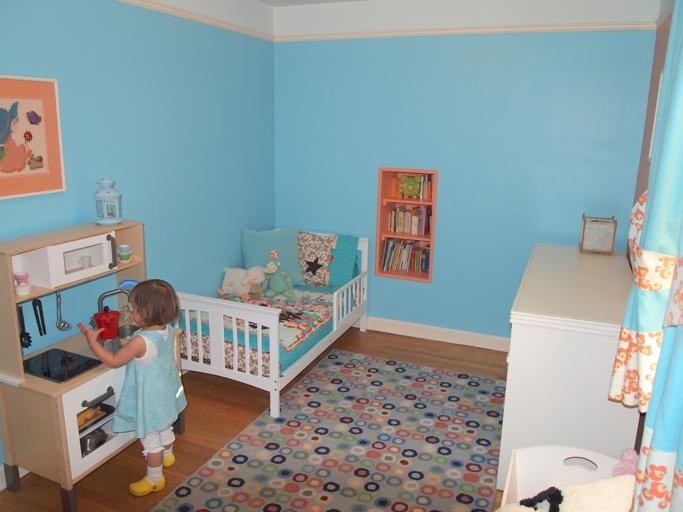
[119,324,141,338]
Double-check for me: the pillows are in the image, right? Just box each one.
[241,228,359,297]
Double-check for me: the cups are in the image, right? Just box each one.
[13,271,32,296]
[115,243,133,263]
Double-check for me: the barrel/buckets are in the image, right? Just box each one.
[93,307,120,340]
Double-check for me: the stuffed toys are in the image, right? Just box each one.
[259,259,293,297]
[218,266,266,300]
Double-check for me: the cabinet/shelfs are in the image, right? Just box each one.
[374,169,439,283]
[0,220,184,512]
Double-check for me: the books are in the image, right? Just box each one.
[381,174,432,273]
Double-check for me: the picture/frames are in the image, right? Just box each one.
[0,76,66,201]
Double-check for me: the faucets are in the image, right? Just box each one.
[98,288,131,311]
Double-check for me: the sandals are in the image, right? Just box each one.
[127,453,177,498]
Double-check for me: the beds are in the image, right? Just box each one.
[166,233,368,418]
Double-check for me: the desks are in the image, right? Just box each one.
[496,241,635,492]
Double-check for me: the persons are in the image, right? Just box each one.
[76,280,188,497]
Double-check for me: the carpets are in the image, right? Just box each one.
[141,349,507,512]
